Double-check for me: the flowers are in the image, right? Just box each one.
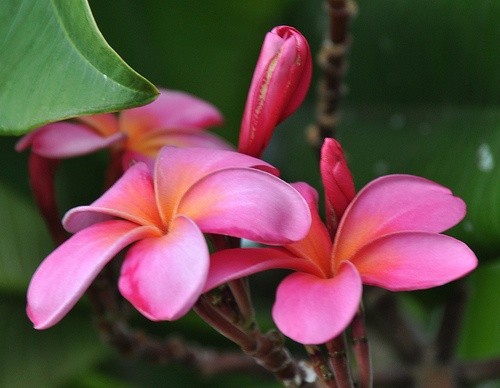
[15,26,479,387]
